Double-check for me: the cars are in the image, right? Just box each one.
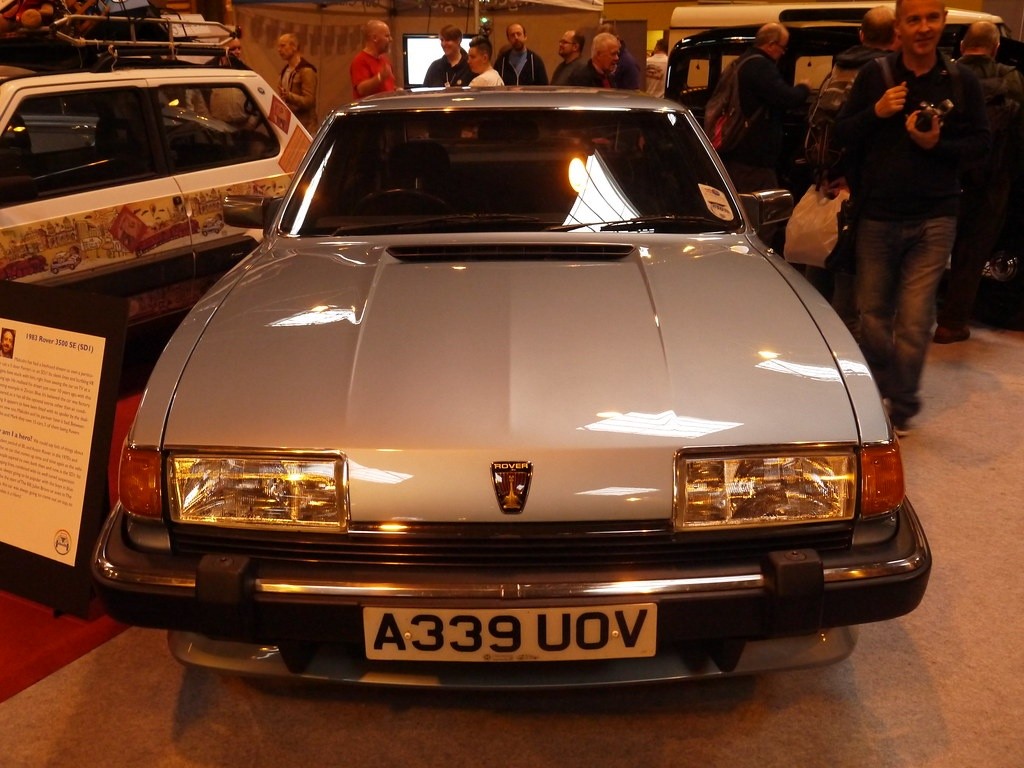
[657,2,1024,330]
[2,1,318,396]
[88,80,935,698]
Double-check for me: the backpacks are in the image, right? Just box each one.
[803,61,874,178]
[699,55,775,153]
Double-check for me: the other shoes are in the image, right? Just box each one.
[933,323,971,346]
[890,414,912,438]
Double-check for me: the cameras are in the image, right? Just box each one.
[914,102,944,134]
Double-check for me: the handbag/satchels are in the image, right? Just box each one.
[825,198,855,270]
[781,181,853,269]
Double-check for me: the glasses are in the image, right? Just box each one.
[558,39,577,45]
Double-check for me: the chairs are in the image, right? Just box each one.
[554,149,651,210]
[373,138,452,214]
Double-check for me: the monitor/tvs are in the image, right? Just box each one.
[402,34,478,89]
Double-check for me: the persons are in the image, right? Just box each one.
[932,22,1024,342]
[423,24,479,86]
[806,8,899,300]
[276,33,319,138]
[827,0,990,438]
[731,21,812,245]
[646,39,670,99]
[467,38,505,87]
[550,30,588,85]
[349,18,404,97]
[191,36,261,131]
[493,23,549,85]
[574,25,640,89]
